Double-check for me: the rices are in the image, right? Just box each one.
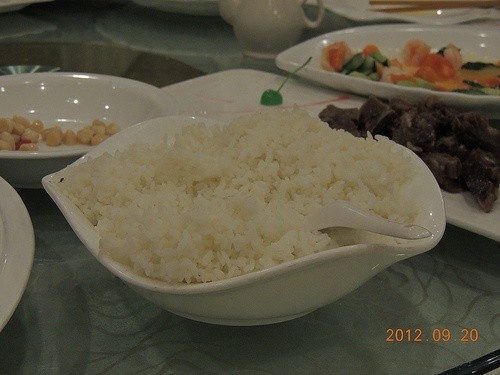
[66,110,417,284]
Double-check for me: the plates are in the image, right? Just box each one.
[307,0,500,25]
[274,23,500,120]
[0,175,35,334]
[0,0,53,14]
[132,0,217,16]
[157,68,500,243]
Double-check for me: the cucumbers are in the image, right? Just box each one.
[339,47,500,96]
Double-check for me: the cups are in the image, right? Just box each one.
[217,0,325,59]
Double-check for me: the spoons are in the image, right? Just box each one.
[307,199,432,240]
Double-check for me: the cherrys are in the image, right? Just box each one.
[261,57,311,105]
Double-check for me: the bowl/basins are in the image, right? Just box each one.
[0,71,165,188]
[40,115,447,327]
